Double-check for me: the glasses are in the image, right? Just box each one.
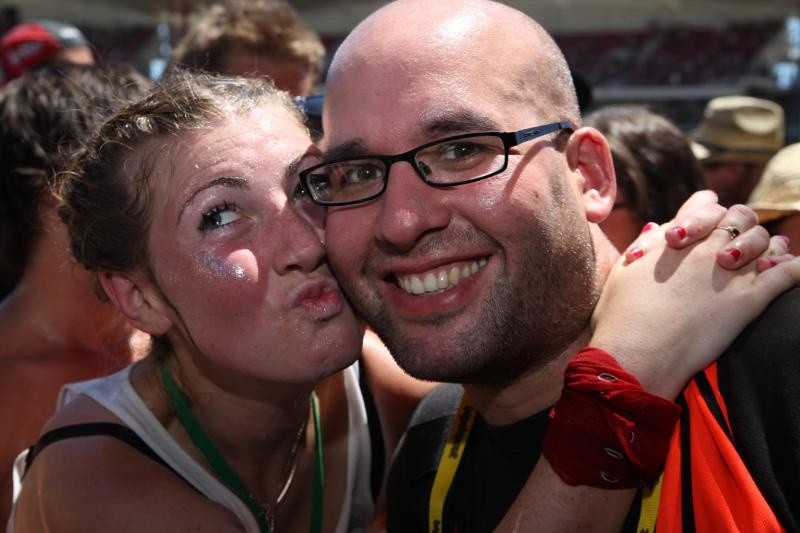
[702,160,728,173]
[293,95,324,133]
[297,120,579,206]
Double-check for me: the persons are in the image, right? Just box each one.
[6,67,800,533]
[318,0,800,533]
[1,0,326,533]
[575,94,799,260]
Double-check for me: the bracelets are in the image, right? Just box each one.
[541,345,681,494]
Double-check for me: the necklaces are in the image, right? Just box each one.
[264,414,308,533]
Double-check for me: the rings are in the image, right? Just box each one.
[716,224,742,239]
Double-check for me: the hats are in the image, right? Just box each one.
[688,97,785,161]
[0,21,100,90]
[742,141,800,225]
